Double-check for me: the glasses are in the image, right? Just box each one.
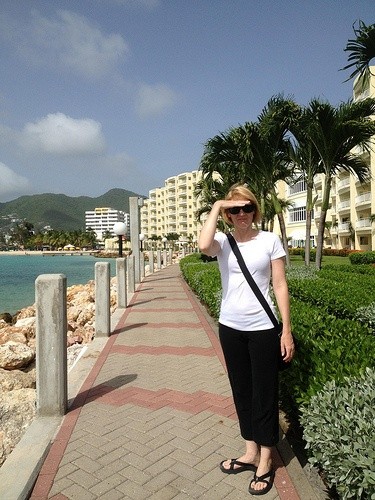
[228,205,254,214]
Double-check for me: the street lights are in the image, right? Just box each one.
[138,234,146,252]
[161,238,168,250]
[113,221,127,258]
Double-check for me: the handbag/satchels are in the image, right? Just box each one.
[277,322,292,371]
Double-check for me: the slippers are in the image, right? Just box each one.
[220,458,258,475]
[248,464,275,495]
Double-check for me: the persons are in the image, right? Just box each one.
[198,184,296,495]
